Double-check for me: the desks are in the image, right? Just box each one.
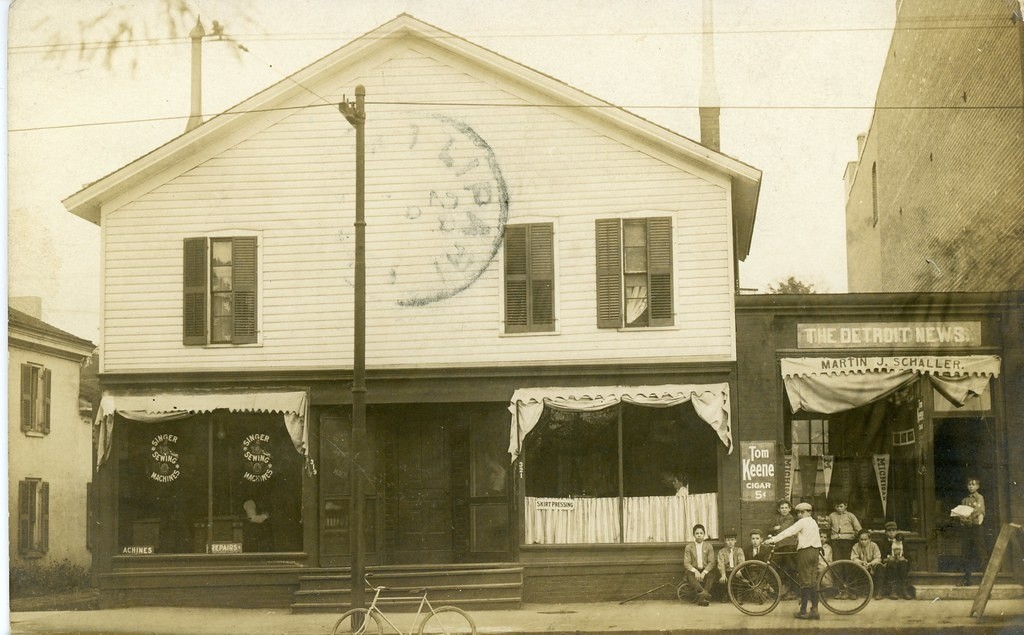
[129,517,183,553]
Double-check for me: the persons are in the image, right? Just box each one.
[957,477,989,586]
[770,499,796,592]
[684,524,715,606]
[717,531,745,605]
[825,498,862,600]
[819,531,833,566]
[673,471,688,496]
[878,521,912,600]
[745,529,776,598]
[238,496,270,524]
[849,528,885,600]
[765,503,822,620]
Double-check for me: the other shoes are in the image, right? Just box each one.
[902,591,911,599]
[808,611,820,620]
[835,590,849,599]
[720,591,728,603]
[876,589,884,600]
[887,591,899,599]
[794,611,807,618]
[957,578,970,586]
[736,593,744,604]
[848,590,856,600]
[696,589,713,605]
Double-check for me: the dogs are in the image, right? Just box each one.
[890,532,905,559]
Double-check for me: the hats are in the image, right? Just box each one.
[884,521,898,530]
[723,530,737,538]
[795,502,813,511]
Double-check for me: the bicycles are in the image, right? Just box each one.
[330,573,477,635]
[727,539,874,617]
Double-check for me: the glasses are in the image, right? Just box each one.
[797,509,810,514]
